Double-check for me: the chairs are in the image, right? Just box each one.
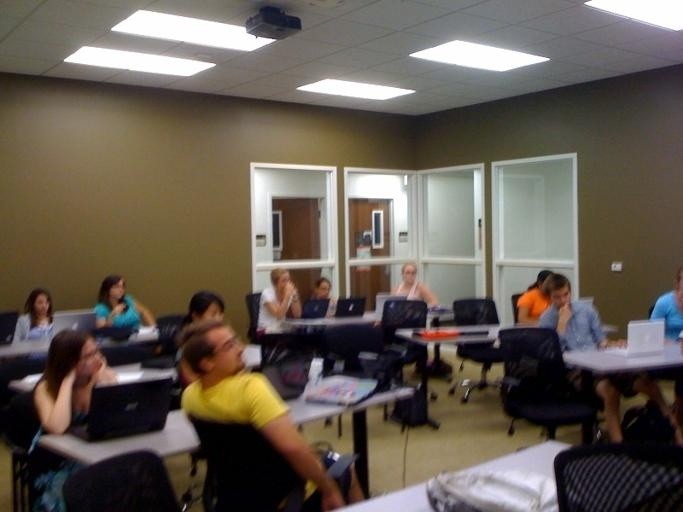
[448,299,498,403]
[187,413,356,512]
[359,300,431,388]
[554,440,682,511]
[155,315,191,340]
[63,451,189,511]
[499,326,604,440]
[246,292,280,363]
[0,311,23,347]
[11,392,39,511]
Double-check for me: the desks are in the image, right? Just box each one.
[0,327,158,365]
[394,324,513,348]
[10,341,263,419]
[332,438,571,512]
[562,342,683,451]
[285,310,451,341]
[40,383,415,494]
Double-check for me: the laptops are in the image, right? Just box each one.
[72,377,174,438]
[610,319,667,355]
[300,298,329,316]
[41,309,99,342]
[333,297,366,317]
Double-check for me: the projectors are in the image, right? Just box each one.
[243,10,304,40]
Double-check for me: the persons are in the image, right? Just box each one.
[27,328,119,511]
[514,269,556,324]
[386,257,439,309]
[93,273,156,332]
[309,275,358,378]
[256,267,303,362]
[10,288,60,344]
[650,266,683,429]
[156,291,225,360]
[539,273,683,447]
[178,318,365,509]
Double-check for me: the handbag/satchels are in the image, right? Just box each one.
[392,387,426,425]
[425,465,557,512]
